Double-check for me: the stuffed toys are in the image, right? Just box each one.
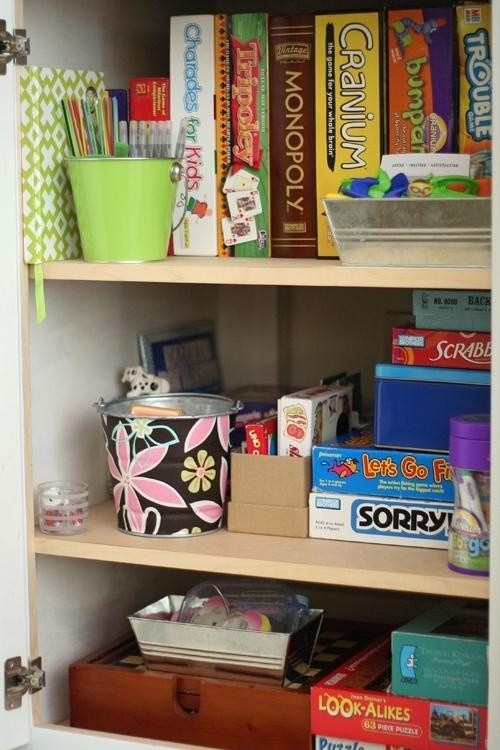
[42,487,82,529]
[189,595,231,628]
[221,609,272,632]
[121,365,172,398]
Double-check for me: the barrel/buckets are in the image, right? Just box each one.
[62,155,190,264]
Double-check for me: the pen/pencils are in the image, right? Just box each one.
[147,120,158,157]
[60,97,118,157]
[139,120,150,157]
[458,469,489,534]
[119,120,128,159]
[129,120,138,158]
[157,120,172,157]
[175,119,188,158]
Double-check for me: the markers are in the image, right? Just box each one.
[115,142,128,158]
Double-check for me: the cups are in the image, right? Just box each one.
[38,479,89,535]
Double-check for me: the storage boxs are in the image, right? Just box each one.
[129,594,326,689]
[229,448,310,538]
[372,363,492,453]
[68,617,385,750]
[324,196,490,269]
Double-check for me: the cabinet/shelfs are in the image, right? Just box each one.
[16,0,486,742]
